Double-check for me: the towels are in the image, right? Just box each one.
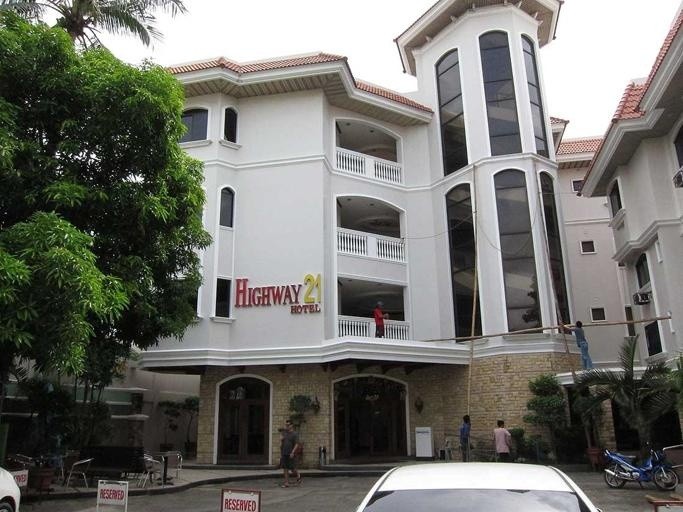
[415,427,435,461]
[319,446,327,465]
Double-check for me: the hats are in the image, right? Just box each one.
[355,461,602,512]
[1,466,21,511]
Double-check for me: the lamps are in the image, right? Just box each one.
[6,450,182,490]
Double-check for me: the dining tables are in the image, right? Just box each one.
[156,394,199,459]
[572,382,606,471]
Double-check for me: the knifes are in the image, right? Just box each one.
[633,291,649,305]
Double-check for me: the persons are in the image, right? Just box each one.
[277,420,304,489]
[374,301,390,338]
[492,419,512,462]
[459,415,471,462]
[564,320,594,370]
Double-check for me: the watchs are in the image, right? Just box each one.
[603,440,678,491]
